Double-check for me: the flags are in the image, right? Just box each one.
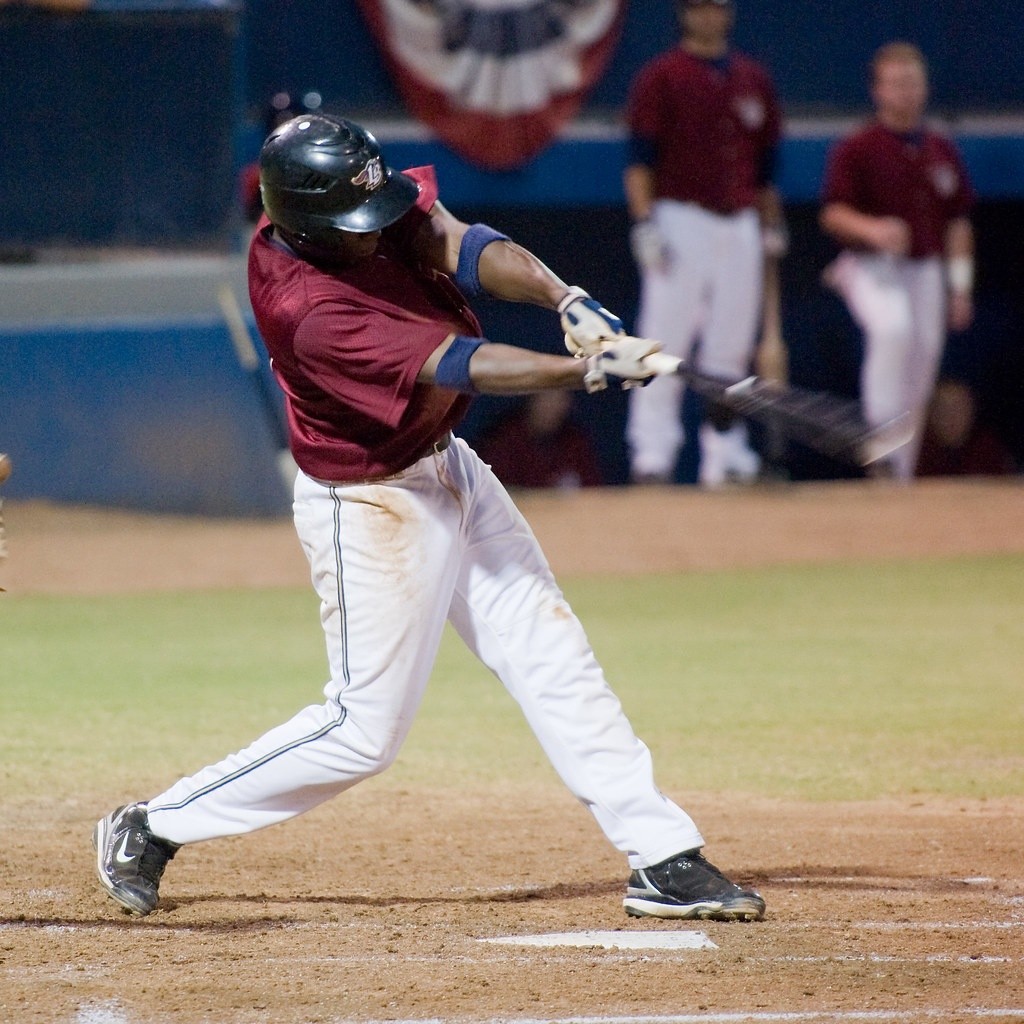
[361,0,622,167]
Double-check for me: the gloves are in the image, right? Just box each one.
[576,330,666,393]
[559,282,627,359]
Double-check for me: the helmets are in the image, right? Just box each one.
[259,113,420,258]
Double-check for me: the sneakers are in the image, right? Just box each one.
[92,802,183,916]
[622,848,766,923]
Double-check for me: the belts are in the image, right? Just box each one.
[420,430,452,458]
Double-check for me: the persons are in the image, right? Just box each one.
[91,116,768,925]
[487,0,1009,496]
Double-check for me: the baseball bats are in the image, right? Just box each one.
[564,333,902,471]
